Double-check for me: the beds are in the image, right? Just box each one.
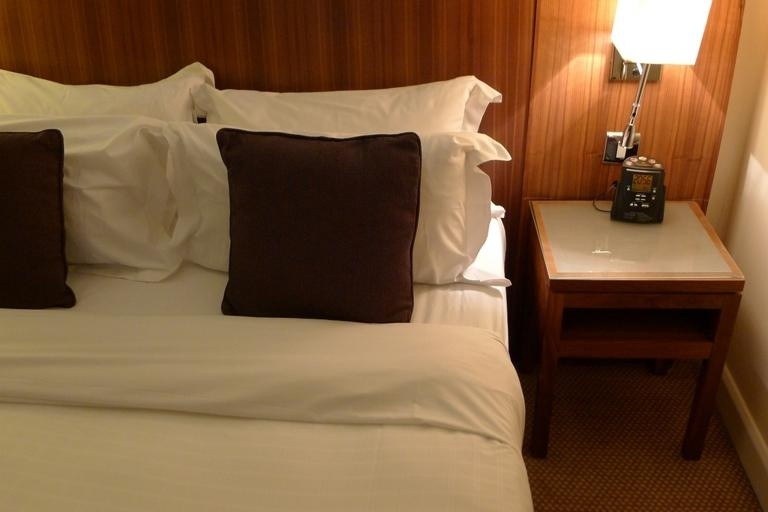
[1,63,532,512]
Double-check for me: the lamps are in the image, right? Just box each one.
[589,0,724,162]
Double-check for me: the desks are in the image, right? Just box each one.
[522,194,745,462]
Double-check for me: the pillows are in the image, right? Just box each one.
[191,72,501,135]
[219,127,419,321]
[163,120,512,290]
[1,60,216,132]
[0,131,74,310]
[0,114,180,285]
